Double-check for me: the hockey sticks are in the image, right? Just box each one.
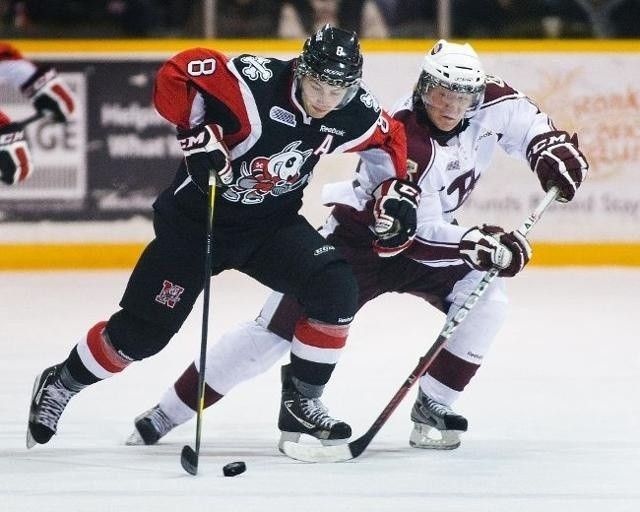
[282,184,559,464]
[181,170,217,475]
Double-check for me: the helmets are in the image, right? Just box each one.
[418,38,488,119]
[294,25,364,111]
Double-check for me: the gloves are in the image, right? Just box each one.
[364,178,421,259]
[175,121,233,198]
[525,130,590,205]
[460,224,533,278]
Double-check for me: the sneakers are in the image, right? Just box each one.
[134,405,178,445]
[29,361,81,444]
[278,391,352,439]
[411,385,469,431]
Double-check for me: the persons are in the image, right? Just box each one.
[25,22,422,446]
[132,39,589,446]
[0,40,76,187]
